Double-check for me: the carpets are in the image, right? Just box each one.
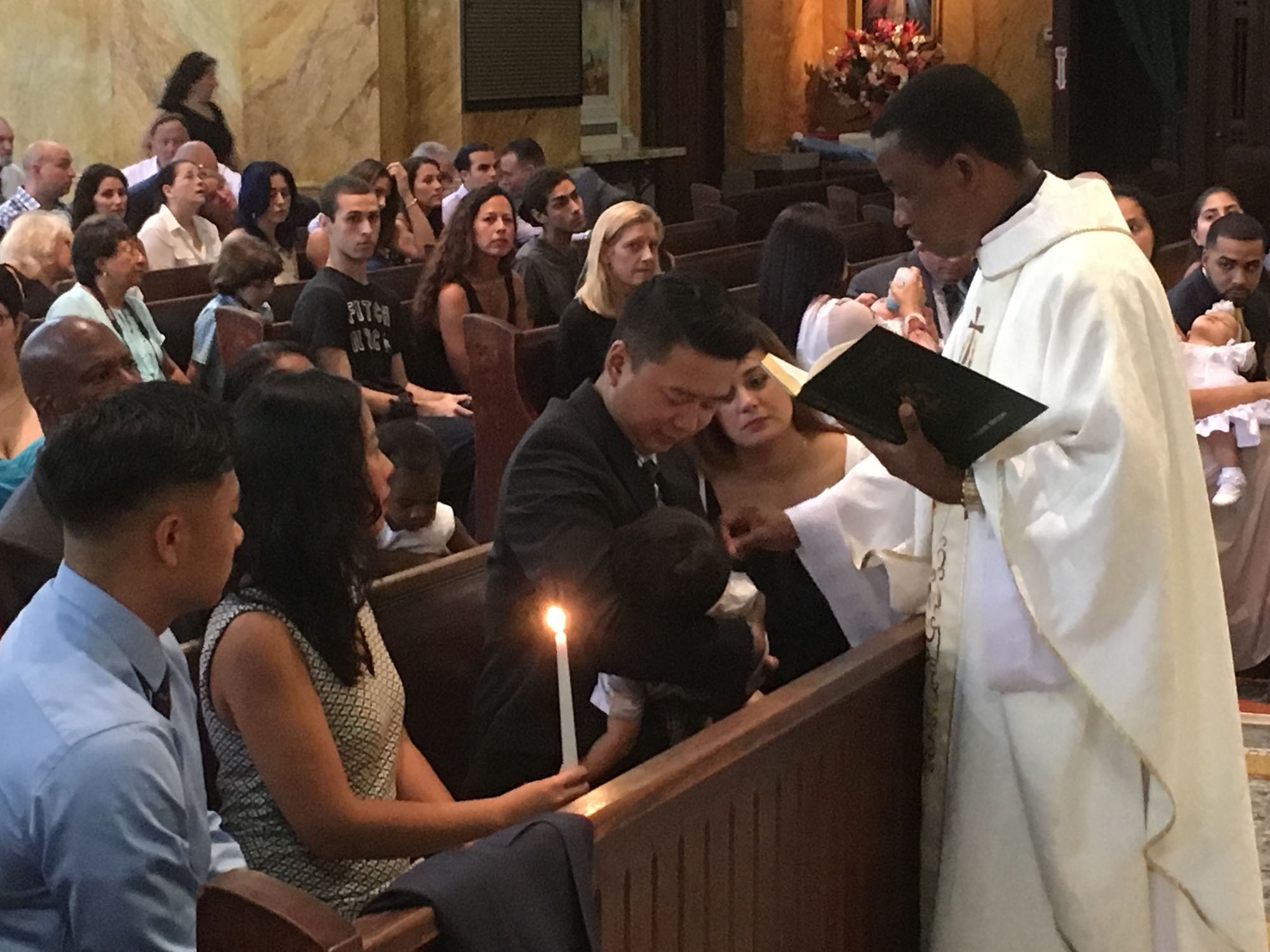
[1238,713,1270,928]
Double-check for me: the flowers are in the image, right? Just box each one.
[813,17,945,103]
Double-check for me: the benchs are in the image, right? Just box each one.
[0,155,1197,952]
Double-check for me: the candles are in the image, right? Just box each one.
[556,632,578,773]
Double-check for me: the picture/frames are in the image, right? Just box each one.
[855,0,942,43]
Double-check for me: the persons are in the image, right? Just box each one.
[751,201,941,364]
[849,234,975,349]
[0,380,250,952]
[194,367,589,922]
[140,52,247,174]
[1071,169,1270,508]
[718,60,1269,952]
[1,116,678,655]
[482,278,857,797]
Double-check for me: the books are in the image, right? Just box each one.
[757,322,1050,476]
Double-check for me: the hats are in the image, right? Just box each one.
[606,507,732,625]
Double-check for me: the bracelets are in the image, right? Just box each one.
[406,199,417,212]
[961,466,985,519]
[900,312,927,332]
